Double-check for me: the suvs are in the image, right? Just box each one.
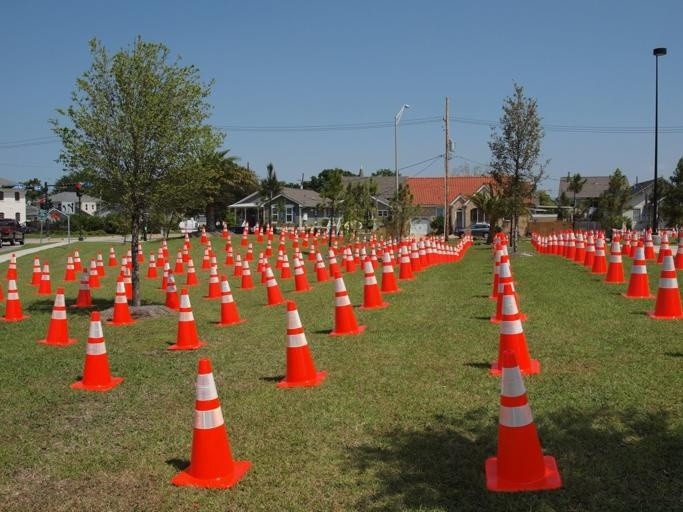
[454,222,492,238]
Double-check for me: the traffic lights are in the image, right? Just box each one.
[76,181,84,197]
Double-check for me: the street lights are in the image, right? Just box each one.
[653,47,667,235]
[394,104,411,202]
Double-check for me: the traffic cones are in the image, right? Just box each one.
[144,254,157,279]
[600,237,628,284]
[620,232,630,256]
[104,275,135,325]
[2,254,19,281]
[73,251,82,272]
[96,251,106,278]
[167,289,205,351]
[620,241,656,299]
[490,232,526,325]
[484,352,562,494]
[171,358,252,489]
[654,231,673,264]
[530,229,607,267]
[290,255,313,294]
[217,276,246,327]
[29,255,42,287]
[674,243,683,269]
[206,261,221,300]
[107,247,118,267]
[263,263,289,307]
[489,283,540,375]
[645,248,683,319]
[69,268,97,308]
[645,230,656,260]
[163,270,180,311]
[277,300,326,388]
[120,249,133,300]
[36,286,79,348]
[36,261,52,295]
[137,243,144,263]
[89,257,101,289]
[68,311,126,393]
[63,254,76,282]
[238,257,255,290]
[159,260,170,290]
[1,275,32,322]
[590,232,608,275]
[628,231,639,258]
[329,270,367,335]
[612,227,677,245]
[156,220,473,286]
[380,248,401,293]
[358,256,390,310]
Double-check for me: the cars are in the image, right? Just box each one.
[0,218,24,247]
